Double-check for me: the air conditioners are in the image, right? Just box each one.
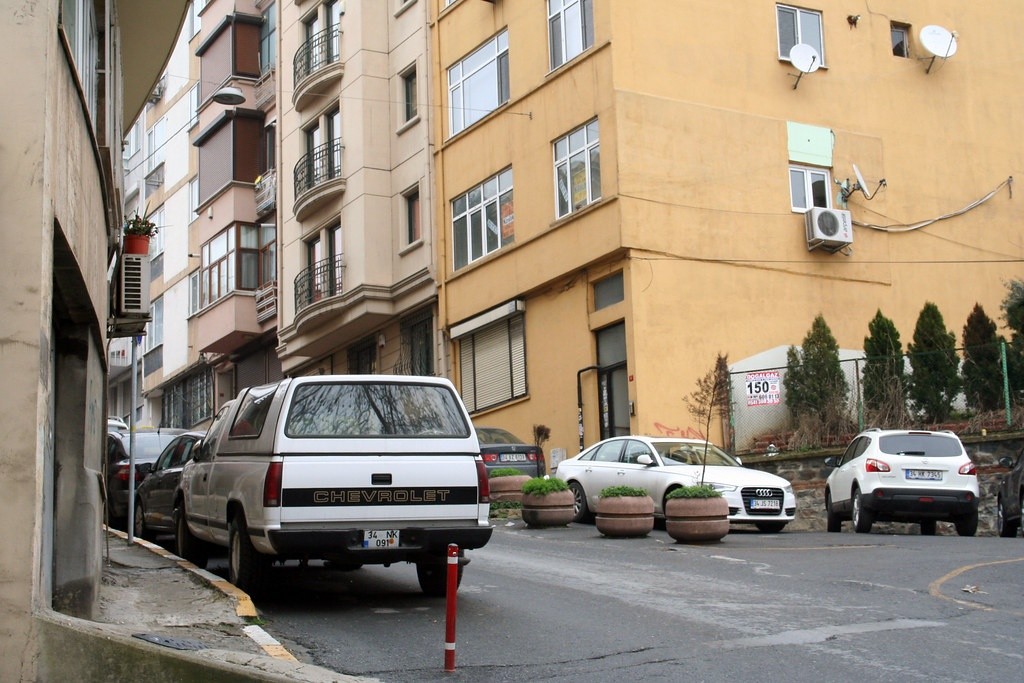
[804,206,853,247]
[110,253,151,333]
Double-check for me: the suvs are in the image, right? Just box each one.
[178,372,495,599]
[824,427,980,537]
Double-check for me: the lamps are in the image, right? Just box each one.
[212,87,247,105]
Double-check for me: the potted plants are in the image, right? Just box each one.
[488,468,532,504]
[665,482,730,544]
[595,486,655,537]
[521,475,575,527]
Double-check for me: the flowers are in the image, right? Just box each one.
[123,200,159,238]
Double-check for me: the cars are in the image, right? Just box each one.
[134,430,208,545]
[555,435,798,534]
[997,446,1024,538]
[473,426,546,480]
[104,428,210,531]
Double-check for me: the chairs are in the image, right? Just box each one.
[671,450,688,462]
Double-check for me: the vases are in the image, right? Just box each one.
[123,234,150,254]
